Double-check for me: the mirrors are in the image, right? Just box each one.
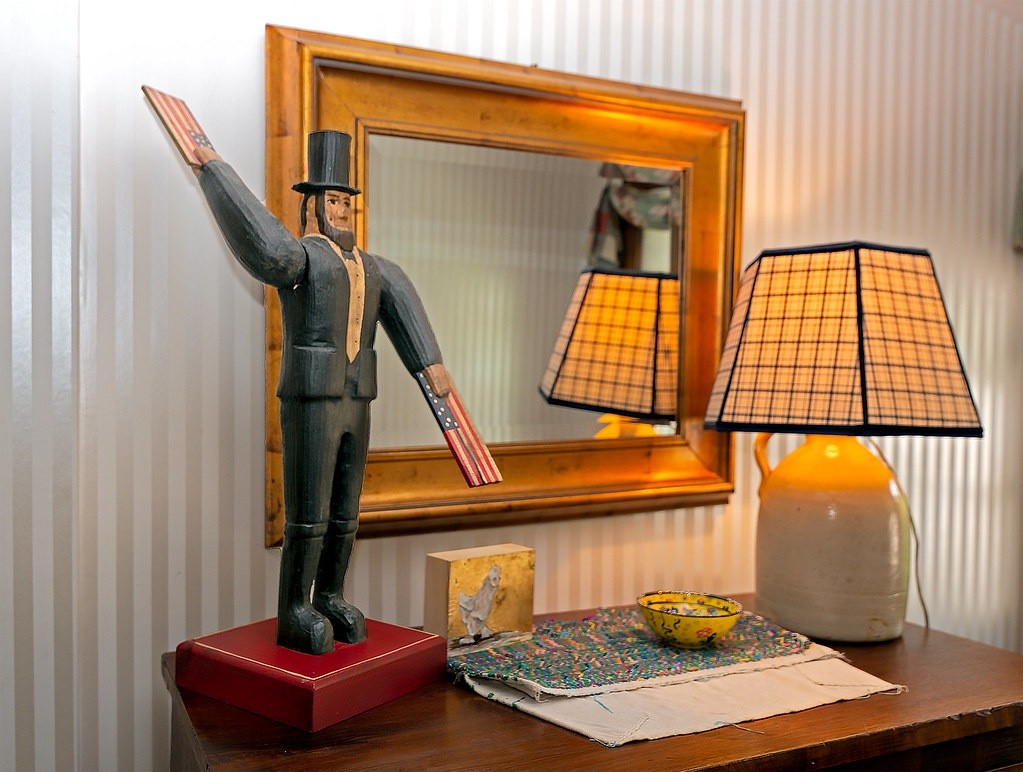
[264,23,747,547]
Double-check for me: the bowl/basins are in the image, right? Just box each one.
[637,591,744,648]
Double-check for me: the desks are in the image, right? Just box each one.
[159,592,1022,772]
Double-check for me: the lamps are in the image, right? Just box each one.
[538,266,683,439]
[704,240,984,645]
